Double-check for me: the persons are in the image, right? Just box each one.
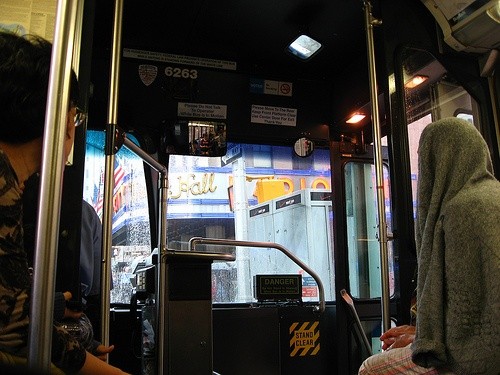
[194,133,218,155]
[79,200,113,297]
[22,163,114,363]
[0,31,131,375]
[358,117,500,375]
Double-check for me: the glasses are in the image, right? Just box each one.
[74,105,87,128]
